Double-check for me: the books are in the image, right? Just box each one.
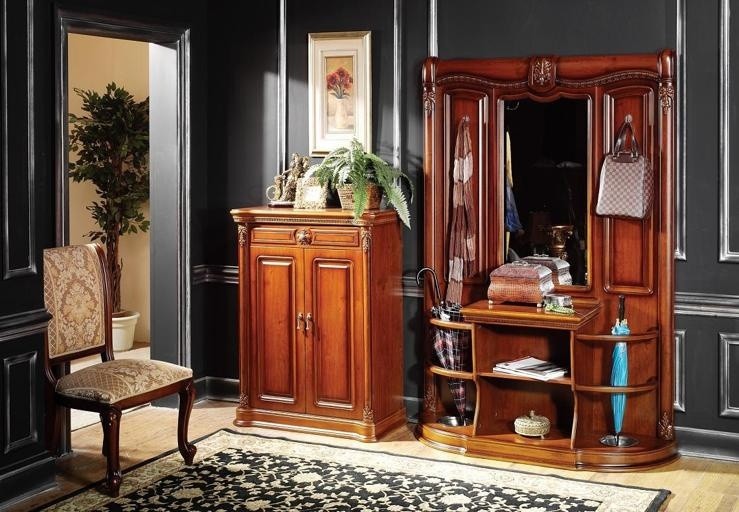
[493,355,568,383]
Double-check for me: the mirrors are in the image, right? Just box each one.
[497,91,596,293]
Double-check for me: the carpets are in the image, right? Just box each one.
[30,429,673,511]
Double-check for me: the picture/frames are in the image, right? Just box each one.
[308,31,372,158]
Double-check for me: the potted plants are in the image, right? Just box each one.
[68,81,149,350]
[300,136,415,230]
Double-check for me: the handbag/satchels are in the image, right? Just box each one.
[596,122,655,219]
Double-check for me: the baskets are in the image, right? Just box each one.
[336,184,383,209]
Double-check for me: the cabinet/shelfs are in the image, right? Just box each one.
[414,48,681,473]
[230,206,406,443]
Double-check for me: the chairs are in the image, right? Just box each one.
[41,242,197,499]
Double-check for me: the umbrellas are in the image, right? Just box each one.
[416,267,466,426]
[610,294,629,445]
